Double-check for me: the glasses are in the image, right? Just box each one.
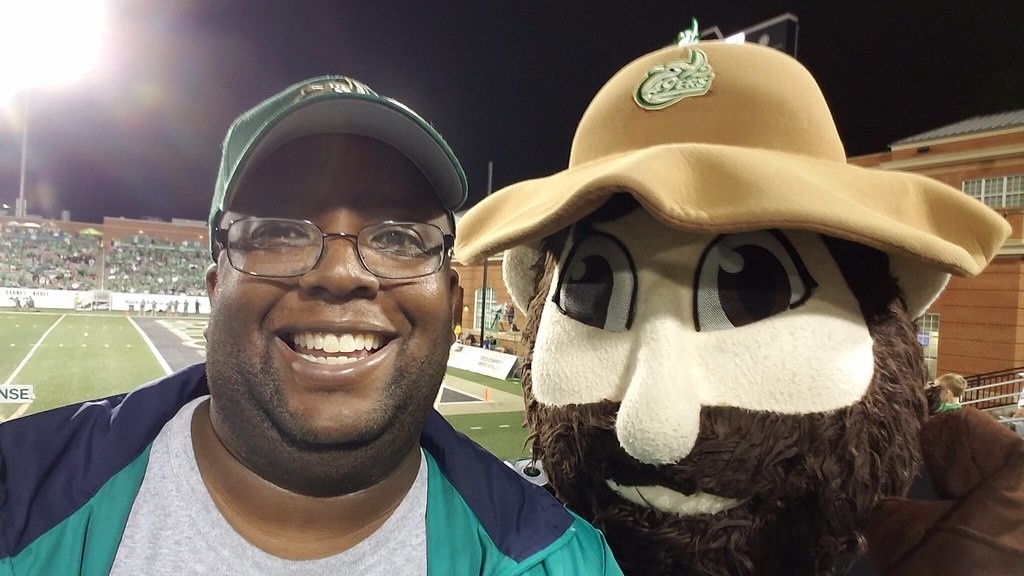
[214,207,457,279]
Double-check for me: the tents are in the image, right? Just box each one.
[7,222,105,239]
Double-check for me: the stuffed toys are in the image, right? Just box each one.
[451,41,1024,576]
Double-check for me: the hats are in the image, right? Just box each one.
[453,15,1014,278]
[207,75,471,265]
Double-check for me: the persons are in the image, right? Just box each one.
[1012,387,1024,418]
[466,332,497,351]
[933,374,967,411]
[1,229,215,317]
[0,76,627,576]
